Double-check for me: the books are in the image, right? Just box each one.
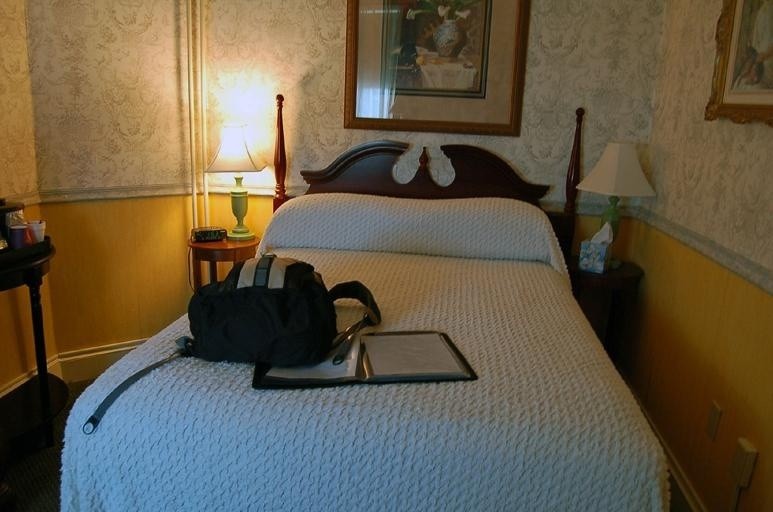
[252,330,479,389]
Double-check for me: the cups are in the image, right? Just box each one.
[8,221,46,250]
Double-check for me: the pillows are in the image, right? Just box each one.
[255,192,566,273]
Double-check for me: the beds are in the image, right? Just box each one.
[60,140,670,512]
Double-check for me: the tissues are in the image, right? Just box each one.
[579,222,613,275]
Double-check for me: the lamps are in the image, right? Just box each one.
[204,124,269,241]
[576,143,657,269]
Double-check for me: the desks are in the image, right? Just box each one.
[569,256,644,365]
[0,236,69,447]
[189,236,260,294]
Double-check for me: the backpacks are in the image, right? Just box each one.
[188,253,382,369]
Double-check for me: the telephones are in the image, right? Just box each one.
[192,226,228,241]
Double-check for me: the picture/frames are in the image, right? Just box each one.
[704,1,773,127]
[344,1,529,136]
